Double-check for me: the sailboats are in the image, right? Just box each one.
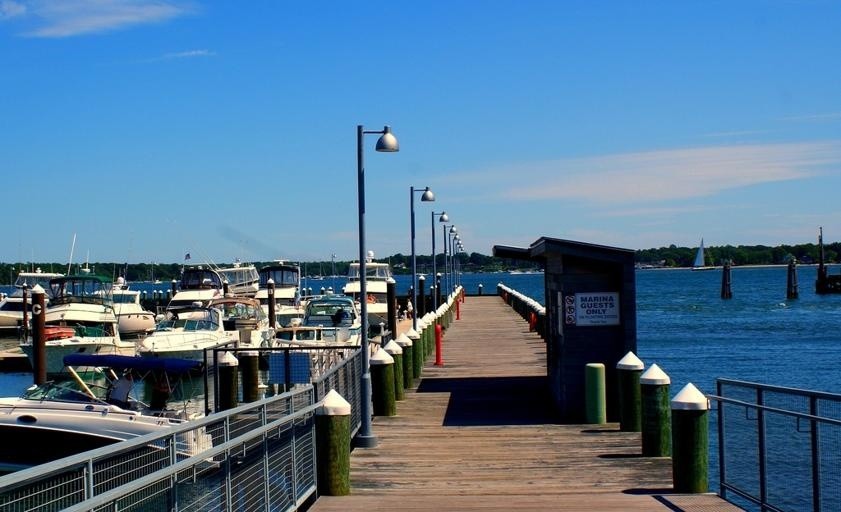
[691,236,718,271]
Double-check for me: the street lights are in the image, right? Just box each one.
[440,224,466,304]
[353,119,400,450]
[409,182,436,332]
[430,210,447,312]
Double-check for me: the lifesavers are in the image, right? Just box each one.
[44,327,76,340]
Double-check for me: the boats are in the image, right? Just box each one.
[1,233,413,491]
[814,224,840,296]
